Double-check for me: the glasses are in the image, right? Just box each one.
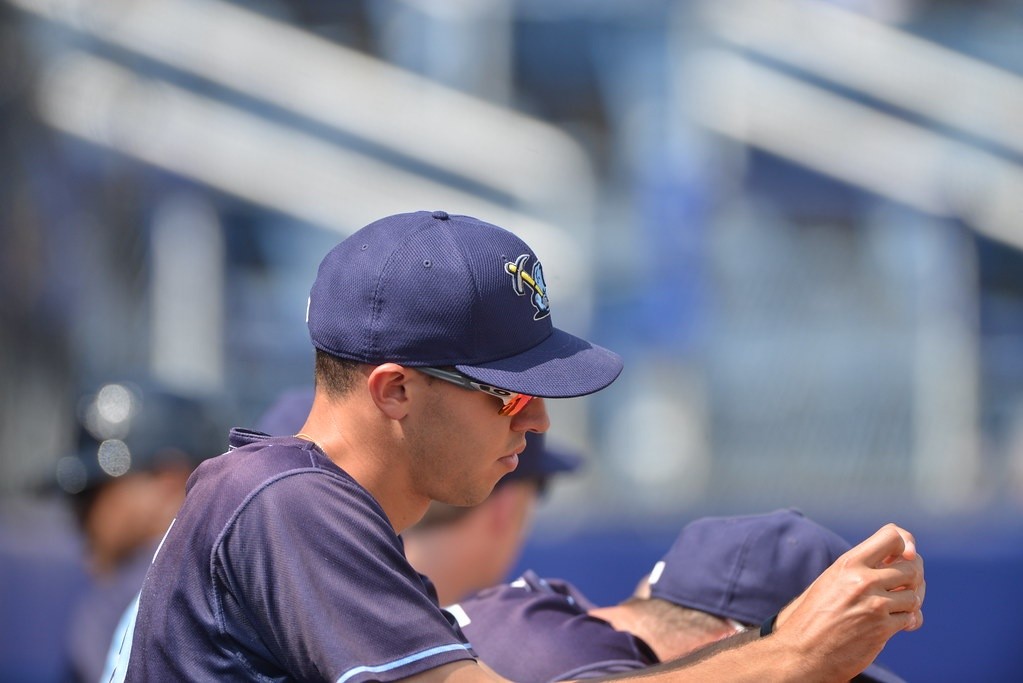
[414,365,531,417]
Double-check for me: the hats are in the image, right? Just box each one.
[305,210,624,398]
[499,429,580,475]
[645,508,908,683]
[74,391,221,480]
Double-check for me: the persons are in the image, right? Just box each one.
[443,506,906,683]
[400,434,580,608]
[100,211,926,683]
[1,378,315,683]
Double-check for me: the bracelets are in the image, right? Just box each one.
[760,614,776,637]
